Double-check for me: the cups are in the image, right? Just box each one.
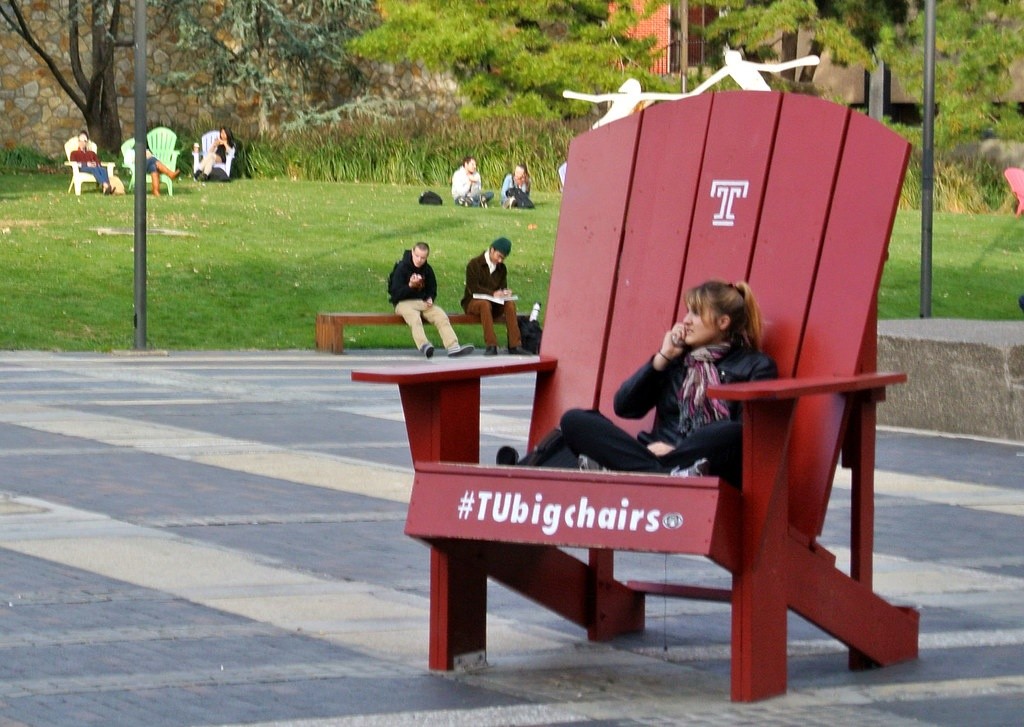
[193,143,199,153]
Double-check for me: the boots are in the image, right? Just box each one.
[157,161,181,180]
[151,171,162,197]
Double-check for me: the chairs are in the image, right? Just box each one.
[191,130,236,177]
[64,136,115,196]
[350,90,918,705]
[128,127,181,196]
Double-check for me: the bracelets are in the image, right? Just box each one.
[659,349,671,363]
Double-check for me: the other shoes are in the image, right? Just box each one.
[508,347,534,355]
[102,184,116,196]
[484,345,498,355]
[200,174,207,183]
[193,169,202,180]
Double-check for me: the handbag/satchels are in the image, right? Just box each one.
[505,187,534,209]
[516,427,578,469]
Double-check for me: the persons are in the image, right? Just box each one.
[124,137,180,195]
[192,126,234,180]
[461,237,531,354]
[450,156,493,208]
[500,163,534,210]
[388,242,475,358]
[70,131,117,196]
[494,279,776,489]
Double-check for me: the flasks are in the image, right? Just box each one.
[529,301,541,322]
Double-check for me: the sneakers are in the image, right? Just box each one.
[447,343,475,357]
[576,452,609,471]
[423,343,434,359]
[668,456,711,477]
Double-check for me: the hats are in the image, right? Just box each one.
[490,237,512,256]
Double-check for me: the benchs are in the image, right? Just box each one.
[315,311,531,355]
